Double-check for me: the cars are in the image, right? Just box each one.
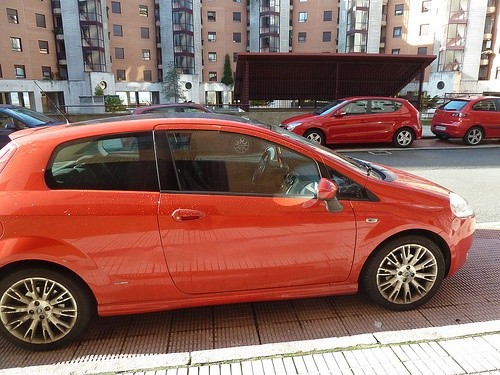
[0,110,478,354]
[95,102,264,156]
[430,95,500,145]
[275,94,423,148]
[0,104,67,148]
